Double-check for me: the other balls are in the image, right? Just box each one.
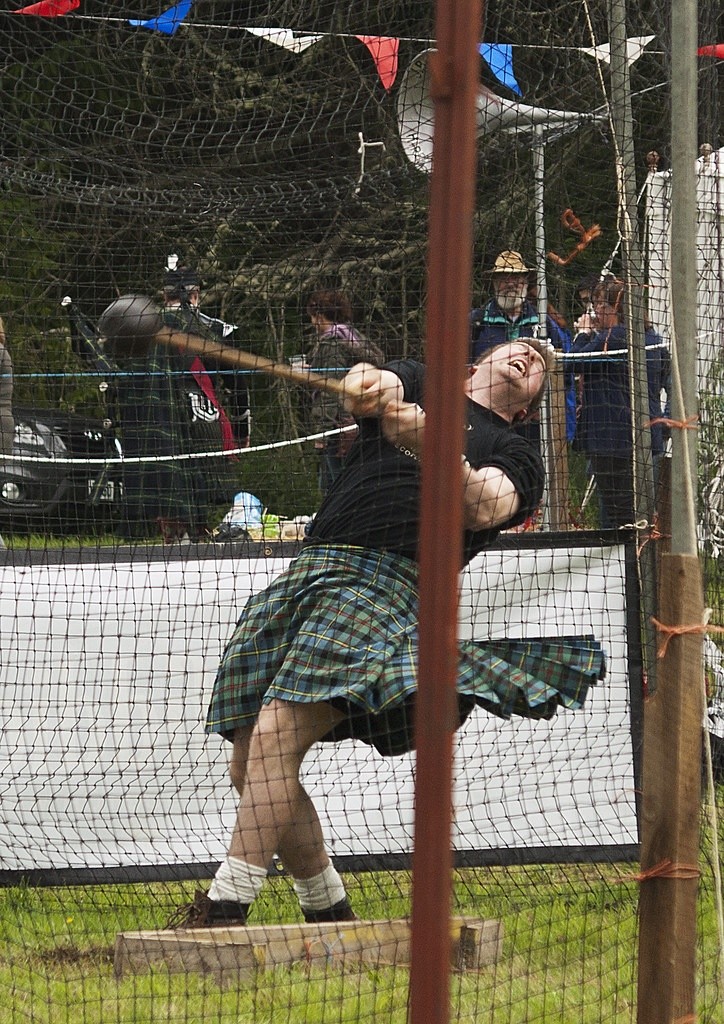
[99,293,370,400]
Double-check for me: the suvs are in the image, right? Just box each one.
[0,405,130,533]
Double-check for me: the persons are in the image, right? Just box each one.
[306,292,385,498]
[165,338,610,940]
[116,272,257,543]
[469,251,676,531]
[0,320,14,450]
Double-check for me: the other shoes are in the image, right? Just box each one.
[168,889,250,929]
[302,896,360,924]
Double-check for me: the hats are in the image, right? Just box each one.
[163,267,202,290]
[483,250,536,291]
